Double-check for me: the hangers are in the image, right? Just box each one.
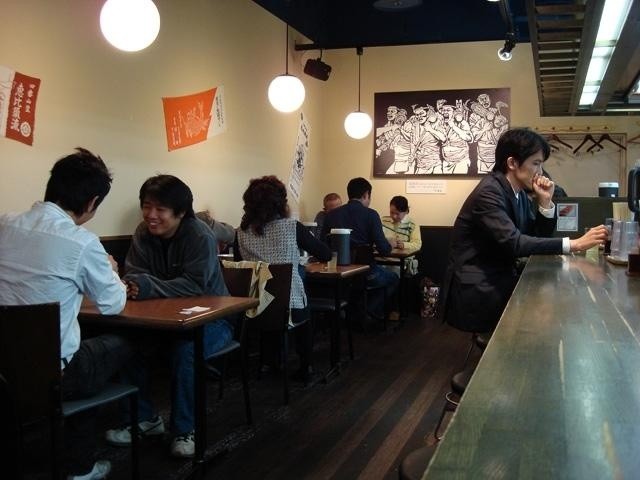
[587,128,626,152]
[548,143,559,150]
[627,134,640,144]
[546,128,572,149]
[572,127,603,154]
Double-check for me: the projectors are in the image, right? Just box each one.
[302,58,333,82]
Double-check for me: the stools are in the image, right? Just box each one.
[435,370,473,442]
[472,335,488,373]
[463,328,487,367]
[397,447,437,480]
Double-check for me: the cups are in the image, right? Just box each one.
[327,252,337,273]
[603,218,614,256]
[610,221,624,257]
[585,228,599,259]
[620,222,640,260]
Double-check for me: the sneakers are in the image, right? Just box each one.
[105,416,165,444]
[171,430,196,458]
[66,459,112,480]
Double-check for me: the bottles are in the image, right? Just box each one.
[301,222,318,237]
[597,182,619,196]
[330,229,351,266]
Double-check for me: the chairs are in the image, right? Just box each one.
[167,268,258,447]
[241,265,311,408]
[309,248,358,367]
[330,244,387,336]
[0,303,139,480]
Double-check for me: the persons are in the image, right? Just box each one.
[374,92,509,175]
[196,209,236,378]
[322,176,399,318]
[232,175,332,384]
[435,128,609,334]
[104,174,235,458]
[314,193,343,238]
[380,196,423,321]
[0,146,128,480]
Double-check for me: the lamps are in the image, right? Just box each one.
[268,24,305,112]
[302,49,333,81]
[496,0,518,62]
[345,45,374,138]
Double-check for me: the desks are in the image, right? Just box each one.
[420,251,640,480]
